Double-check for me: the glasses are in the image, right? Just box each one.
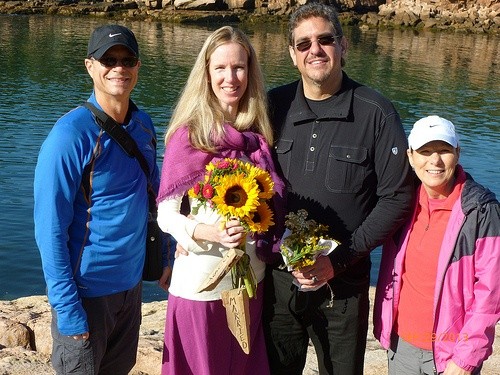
[90,56,138,67]
[294,35,342,52]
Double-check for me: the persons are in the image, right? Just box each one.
[34,25,174,375]
[373,114,500,375]
[174,1,416,375]
[156,25,284,375]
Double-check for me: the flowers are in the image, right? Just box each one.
[188,157,275,299]
[277,208,331,271]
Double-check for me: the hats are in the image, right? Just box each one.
[407,115,459,150]
[87,25,141,61]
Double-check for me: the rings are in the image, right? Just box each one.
[224,229,231,239]
[312,275,318,283]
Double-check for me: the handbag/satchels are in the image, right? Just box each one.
[142,220,168,282]
